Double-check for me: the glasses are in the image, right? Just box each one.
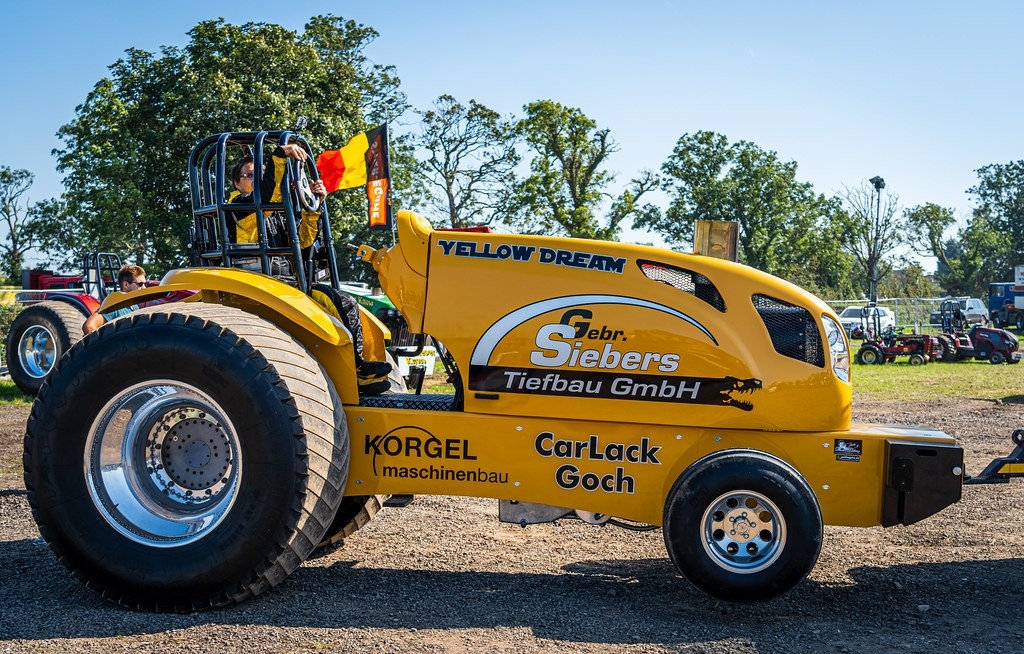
[128,282,149,287]
[240,172,253,178]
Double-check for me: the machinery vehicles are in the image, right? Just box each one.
[934,299,1024,364]
[24,133,966,616]
[7,251,200,398]
[858,326,939,366]
[322,279,428,387]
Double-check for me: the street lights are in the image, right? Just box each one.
[869,176,886,307]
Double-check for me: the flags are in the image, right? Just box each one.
[315,123,389,196]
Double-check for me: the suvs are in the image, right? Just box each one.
[930,295,989,326]
[839,303,896,336]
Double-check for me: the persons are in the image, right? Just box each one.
[944,308,970,334]
[863,323,899,348]
[82,265,146,335]
[224,143,391,395]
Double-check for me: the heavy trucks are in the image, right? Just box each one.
[988,265,1024,333]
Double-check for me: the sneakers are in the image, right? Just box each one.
[355,354,392,396]
